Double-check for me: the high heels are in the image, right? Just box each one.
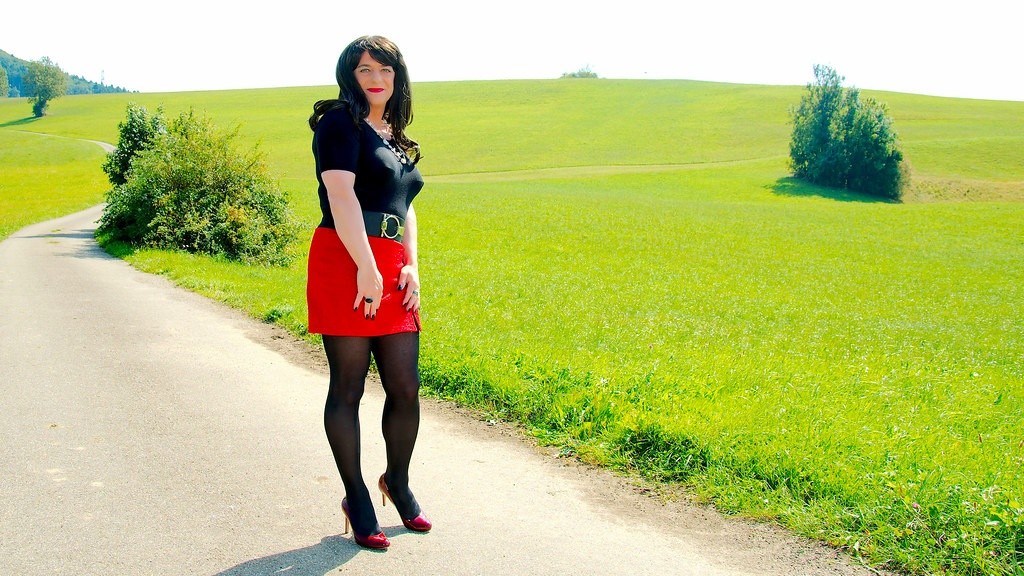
[379,473,432,532]
[340,497,390,549]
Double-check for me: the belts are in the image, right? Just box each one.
[363,212,406,242]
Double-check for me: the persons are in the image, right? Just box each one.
[307,35,432,548]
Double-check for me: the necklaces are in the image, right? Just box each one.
[364,117,407,164]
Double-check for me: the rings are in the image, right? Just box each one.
[413,290,418,296]
[366,298,373,303]
